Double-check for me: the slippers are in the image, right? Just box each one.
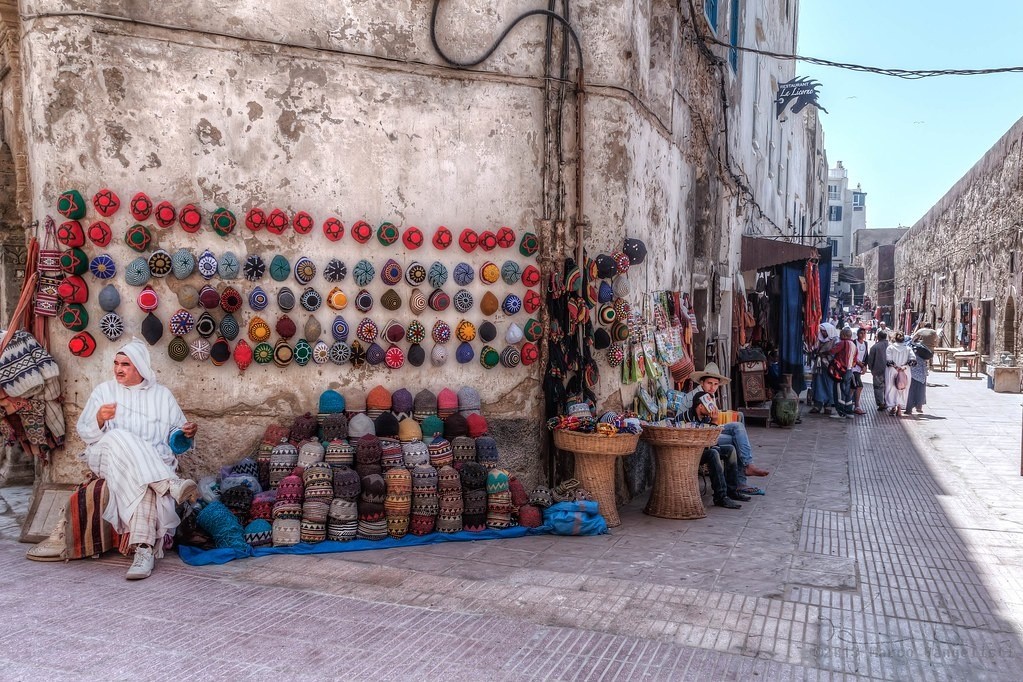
[738,486,765,495]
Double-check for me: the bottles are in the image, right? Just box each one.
[772,383,799,428]
[782,374,799,400]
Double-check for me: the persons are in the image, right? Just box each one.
[806,292,978,419]
[674,362,771,495]
[672,389,751,509]
[76,336,199,581]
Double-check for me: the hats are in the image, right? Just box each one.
[219,384,554,548]
[55,191,647,371]
[689,362,732,386]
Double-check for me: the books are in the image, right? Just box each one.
[698,392,718,414]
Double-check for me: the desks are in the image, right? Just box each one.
[931,346,981,379]
[985,360,1021,393]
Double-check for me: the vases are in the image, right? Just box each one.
[773,374,798,428]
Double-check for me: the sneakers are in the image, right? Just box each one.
[126,544,155,580]
[714,496,742,509]
[169,479,198,506]
[727,491,752,501]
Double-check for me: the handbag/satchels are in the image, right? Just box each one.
[622,291,698,382]
[827,359,847,383]
[912,342,933,360]
[895,369,909,391]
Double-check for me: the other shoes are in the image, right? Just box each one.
[876,404,923,416]
[824,408,832,414]
[809,406,820,414]
[846,413,855,419]
[853,406,867,414]
[830,412,846,419]
